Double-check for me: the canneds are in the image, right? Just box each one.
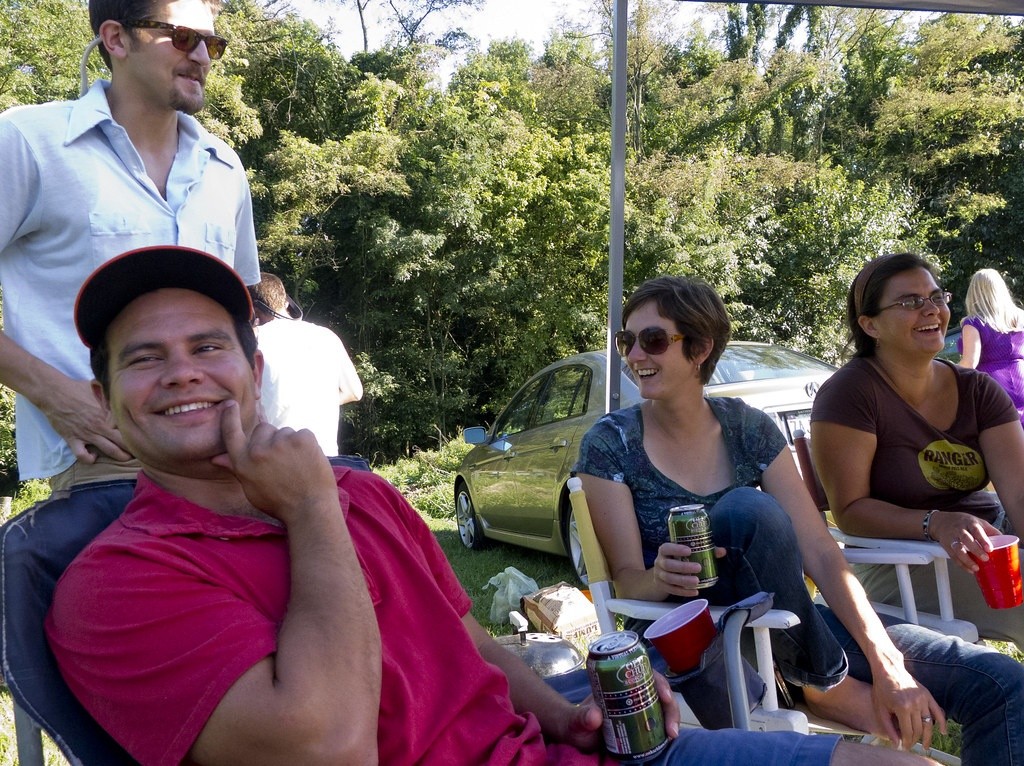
[587,631,669,764]
[668,503,719,589]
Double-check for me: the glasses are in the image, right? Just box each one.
[123,19,228,61]
[877,290,954,313]
[614,328,686,356]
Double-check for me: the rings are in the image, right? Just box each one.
[921,716,932,723]
[951,541,959,549]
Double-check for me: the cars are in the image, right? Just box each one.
[454,341,849,591]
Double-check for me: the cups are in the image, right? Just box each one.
[962,536,1024,609]
[643,599,718,673]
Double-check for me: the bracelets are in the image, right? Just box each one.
[923,510,940,544]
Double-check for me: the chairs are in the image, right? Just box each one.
[566,430,1008,766]
[0,454,372,766]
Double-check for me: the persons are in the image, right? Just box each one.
[0,0,263,492]
[810,253,1024,650]
[245,273,362,457]
[47,248,943,766]
[577,280,1024,766]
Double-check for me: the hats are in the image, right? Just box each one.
[74,244,255,348]
